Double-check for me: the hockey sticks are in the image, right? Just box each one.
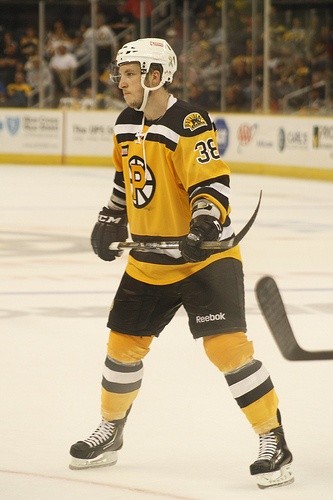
[255,275,333,362]
[108,189,263,251]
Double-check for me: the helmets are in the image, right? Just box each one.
[115,37,178,92]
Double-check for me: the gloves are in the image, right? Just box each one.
[90,206,129,262]
[178,214,224,264]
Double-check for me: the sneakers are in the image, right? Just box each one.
[67,417,129,471]
[248,423,296,490]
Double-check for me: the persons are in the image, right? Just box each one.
[0,0,333,115]
[69,38,294,487]
